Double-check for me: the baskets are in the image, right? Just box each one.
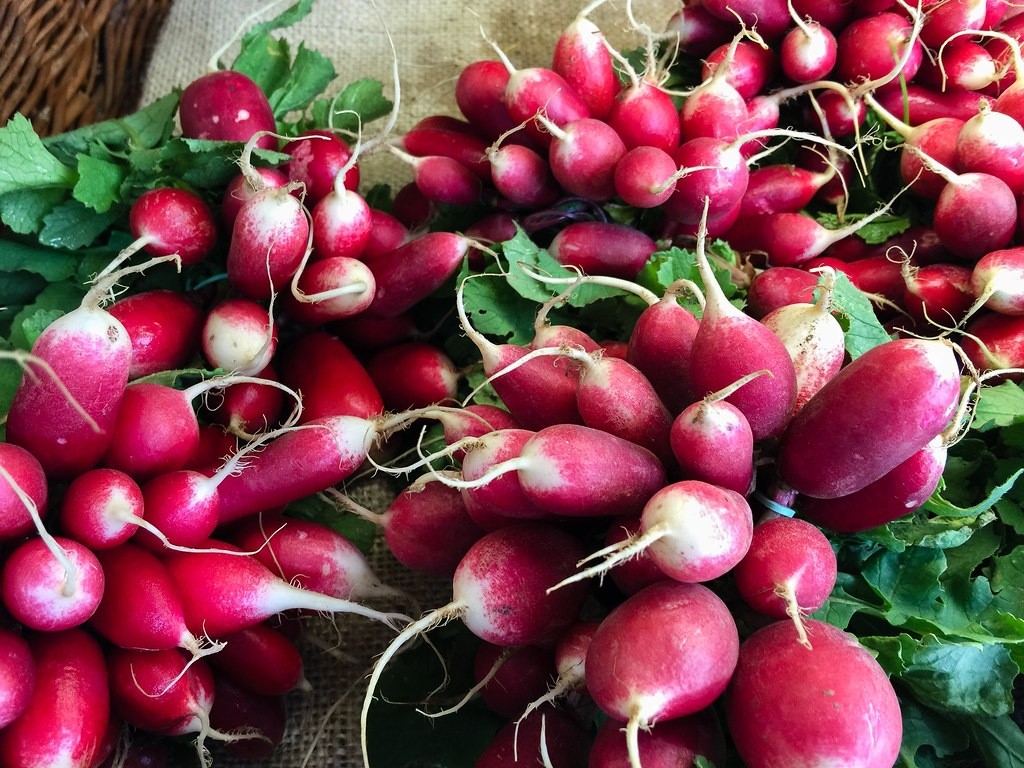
[0,0,173,141]
